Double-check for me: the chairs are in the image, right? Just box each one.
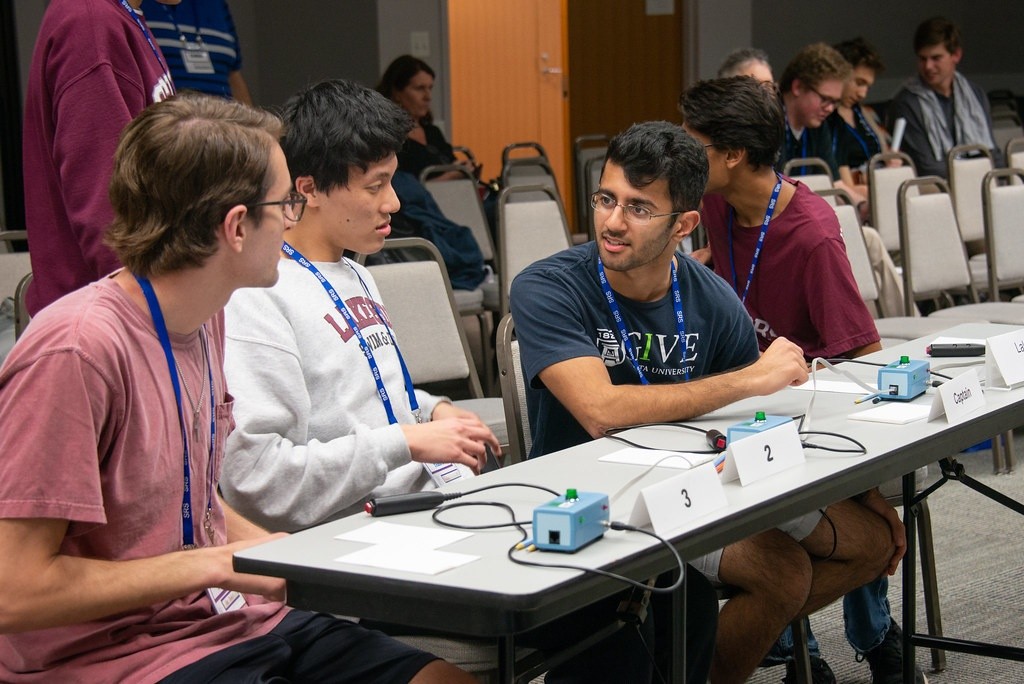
[0,88,1024,684]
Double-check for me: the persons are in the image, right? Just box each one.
[716,47,773,83]
[778,41,922,318]
[0,89,478,684]
[377,54,479,182]
[678,75,930,684]
[511,120,908,684]
[140,0,253,107]
[225,78,537,684]
[890,16,1007,186]
[22,0,177,318]
[826,35,903,169]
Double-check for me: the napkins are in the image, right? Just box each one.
[792,379,878,396]
[848,402,932,424]
[599,448,719,469]
[334,543,482,577]
[333,520,474,549]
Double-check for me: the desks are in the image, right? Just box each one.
[230,320,1024,684]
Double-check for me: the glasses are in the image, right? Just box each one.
[806,81,843,111]
[766,82,779,95]
[591,191,684,225]
[220,190,308,222]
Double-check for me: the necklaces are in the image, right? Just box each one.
[173,329,205,443]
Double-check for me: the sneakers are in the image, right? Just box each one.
[856,616,927,684]
[784,654,837,684]
